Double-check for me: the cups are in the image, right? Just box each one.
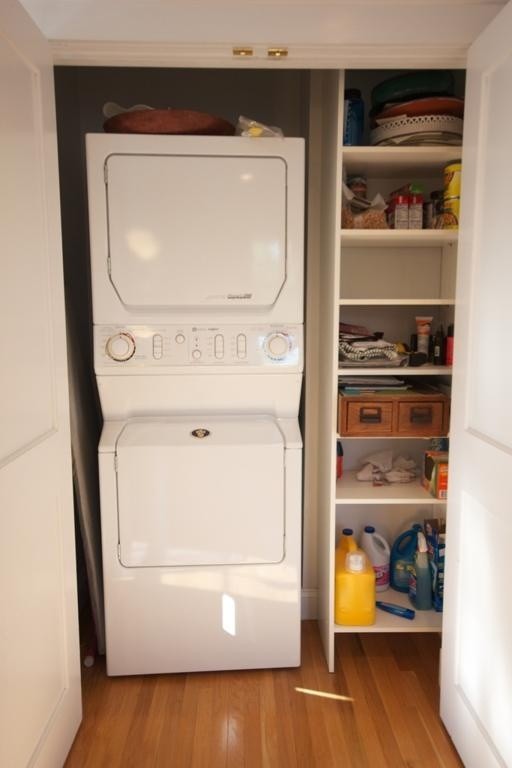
[446,335,454,366]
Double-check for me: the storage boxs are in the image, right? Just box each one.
[338,388,450,438]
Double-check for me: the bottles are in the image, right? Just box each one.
[343,88,364,147]
[433,325,444,366]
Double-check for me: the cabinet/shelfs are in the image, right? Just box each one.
[302,70,467,672]
